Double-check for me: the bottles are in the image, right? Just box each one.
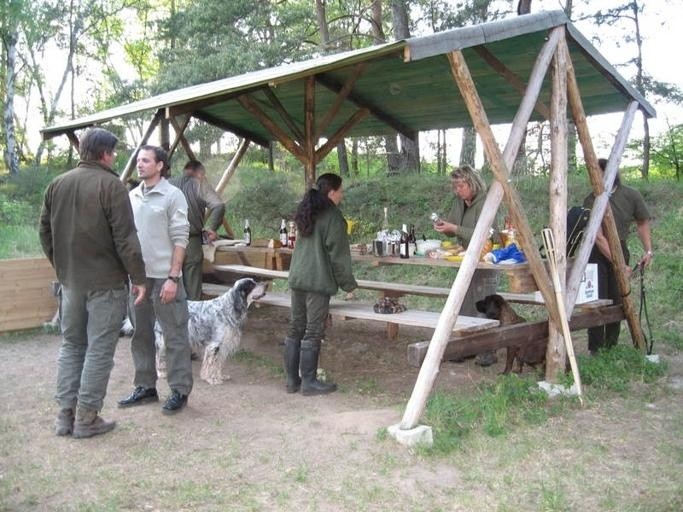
[407,224,415,257]
[398,223,409,258]
[490,228,500,250]
[287,223,296,247]
[381,204,391,236]
[241,217,252,247]
[277,218,289,247]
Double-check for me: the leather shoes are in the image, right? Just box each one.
[119,387,158,407]
[162,391,187,415]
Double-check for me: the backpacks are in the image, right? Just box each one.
[537,204,592,262]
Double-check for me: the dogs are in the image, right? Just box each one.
[120,277,267,385]
[475,294,572,378]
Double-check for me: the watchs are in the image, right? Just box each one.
[169,274,182,283]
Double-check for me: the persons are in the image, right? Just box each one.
[36,125,147,439]
[169,160,226,303]
[581,158,655,359]
[117,147,194,414]
[285,171,358,396]
[432,163,503,367]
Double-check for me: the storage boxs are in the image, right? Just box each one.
[535,258,599,306]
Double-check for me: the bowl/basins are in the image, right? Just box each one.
[414,239,441,255]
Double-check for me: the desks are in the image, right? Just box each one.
[201,238,277,271]
[275,245,569,389]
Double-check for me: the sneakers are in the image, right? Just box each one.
[475,352,498,367]
[54,412,74,436]
[73,417,115,439]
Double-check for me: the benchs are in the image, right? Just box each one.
[215,261,545,339]
[161,272,506,339]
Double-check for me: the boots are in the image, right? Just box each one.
[299,339,337,396]
[283,335,301,393]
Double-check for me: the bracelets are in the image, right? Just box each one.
[647,250,654,257]
